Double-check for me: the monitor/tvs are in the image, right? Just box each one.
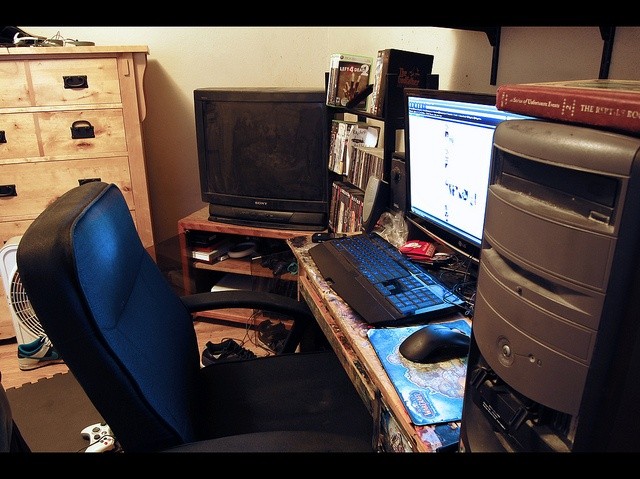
[194,87,348,231]
[405,88,539,266]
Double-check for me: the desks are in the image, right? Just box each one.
[289,233,464,454]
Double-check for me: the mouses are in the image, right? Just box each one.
[399,326,471,362]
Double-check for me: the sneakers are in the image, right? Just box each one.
[202,338,257,367]
[17,335,64,371]
[257,319,289,354]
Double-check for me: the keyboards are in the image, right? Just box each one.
[308,232,467,325]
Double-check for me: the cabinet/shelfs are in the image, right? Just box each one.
[177,202,330,332]
[328,106,407,236]
[1,45,158,345]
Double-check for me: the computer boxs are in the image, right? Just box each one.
[456,120,639,453]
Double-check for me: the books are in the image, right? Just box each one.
[327,180,366,237]
[325,48,435,116]
[327,120,397,196]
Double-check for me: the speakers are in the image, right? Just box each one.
[362,175,388,228]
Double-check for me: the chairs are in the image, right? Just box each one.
[16,183,367,452]
